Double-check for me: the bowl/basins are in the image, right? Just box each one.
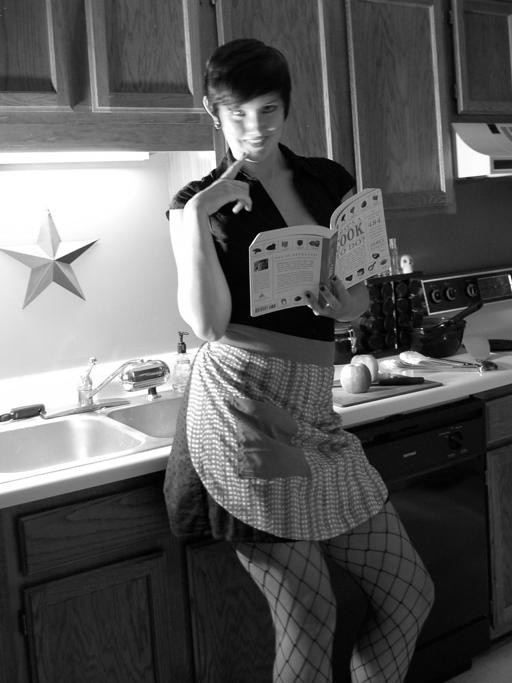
[409,314,465,356]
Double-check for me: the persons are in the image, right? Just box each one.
[163,35,434,682]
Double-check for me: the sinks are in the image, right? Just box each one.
[0,411,146,479]
[106,394,185,441]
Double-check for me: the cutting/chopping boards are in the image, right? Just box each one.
[330,377,444,408]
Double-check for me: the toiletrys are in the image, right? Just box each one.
[171,330,194,393]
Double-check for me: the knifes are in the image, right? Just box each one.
[330,375,424,387]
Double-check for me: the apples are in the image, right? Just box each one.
[340,363,369,393]
[351,354,379,382]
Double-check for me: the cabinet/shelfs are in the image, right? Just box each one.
[476,386,511,641]
[1,469,275,683]
[217,0,457,221]
[452,0,511,124]
[2,0,217,153]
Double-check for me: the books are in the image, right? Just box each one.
[245,182,394,321]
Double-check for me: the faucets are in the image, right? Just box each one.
[78,356,145,407]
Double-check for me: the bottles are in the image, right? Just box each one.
[388,238,400,276]
